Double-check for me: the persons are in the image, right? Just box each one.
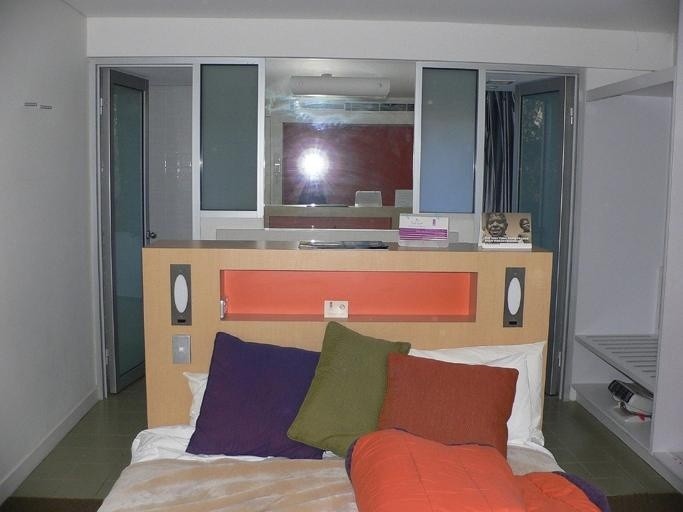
[483,212,509,240]
[293,173,328,204]
[516,217,531,246]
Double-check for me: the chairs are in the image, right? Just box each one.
[354,189,414,208]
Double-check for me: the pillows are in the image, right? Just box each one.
[287,322,411,457]
[185,331,325,460]
[408,345,519,449]
[379,351,519,456]
[184,372,209,427]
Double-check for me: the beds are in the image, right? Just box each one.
[94,238,608,512]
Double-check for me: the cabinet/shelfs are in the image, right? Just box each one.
[570,61,683,496]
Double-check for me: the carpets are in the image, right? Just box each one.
[0,496,104,512]
[608,491,683,511]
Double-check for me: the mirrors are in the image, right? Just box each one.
[264,58,418,229]
